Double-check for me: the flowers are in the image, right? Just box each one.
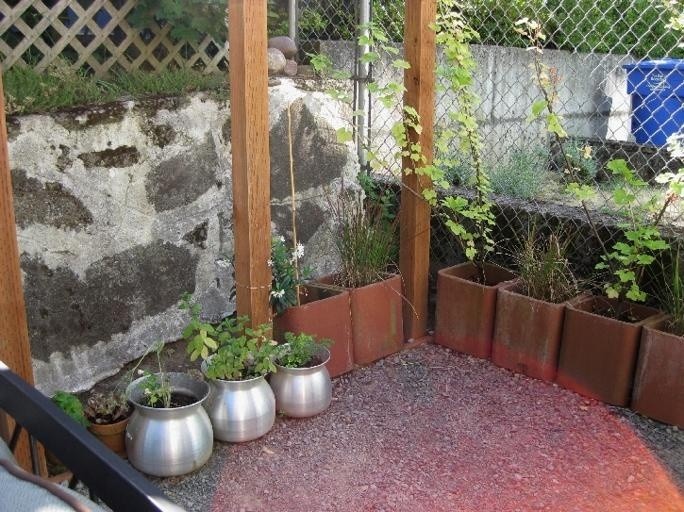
[214,227,307,318]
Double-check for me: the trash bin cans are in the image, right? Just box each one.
[621,57,683,145]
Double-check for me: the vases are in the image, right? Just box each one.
[274,285,350,381]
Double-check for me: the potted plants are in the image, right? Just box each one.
[84,289,333,479]
